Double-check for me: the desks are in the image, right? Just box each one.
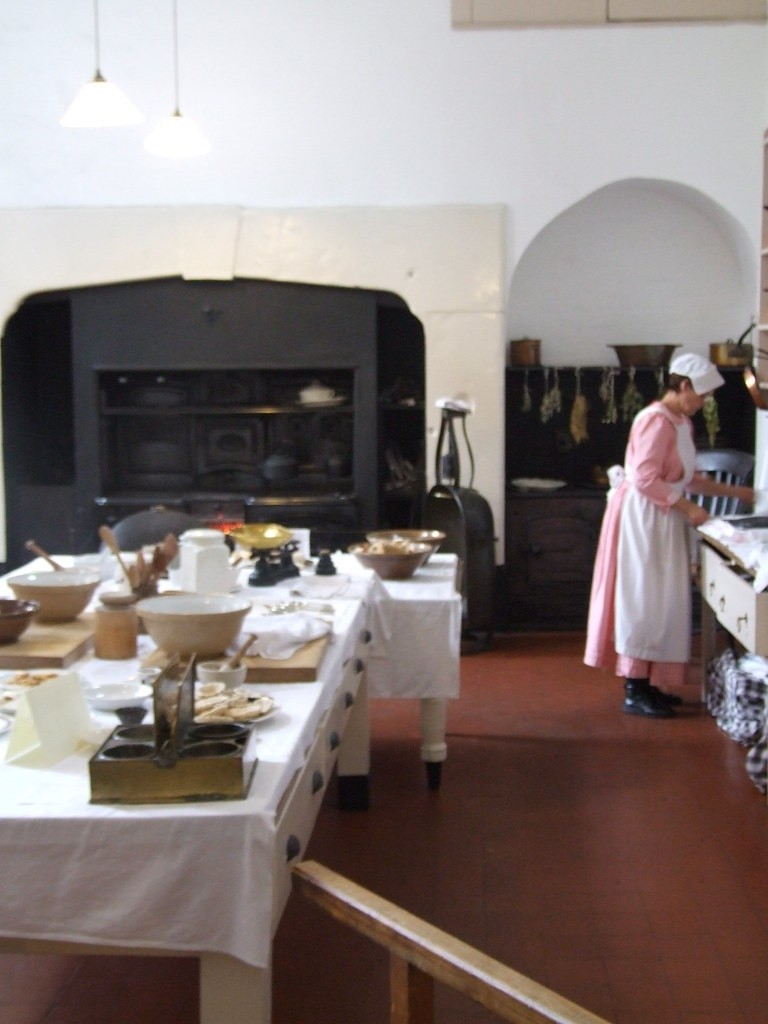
[694,524,767,703]
[0,552,468,1019]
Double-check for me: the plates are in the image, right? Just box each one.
[239,694,283,723]
[511,478,567,493]
[0,668,60,734]
[294,395,347,407]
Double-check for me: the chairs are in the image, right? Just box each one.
[681,450,754,523]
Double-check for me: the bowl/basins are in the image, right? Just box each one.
[0,598,41,643]
[194,661,247,691]
[347,528,447,580]
[135,593,253,662]
[7,570,103,623]
[83,682,154,712]
[135,667,162,683]
[229,523,294,549]
[607,343,684,368]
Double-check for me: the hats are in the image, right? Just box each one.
[669,352,724,395]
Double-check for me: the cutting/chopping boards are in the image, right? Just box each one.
[0,612,98,668]
[138,620,333,686]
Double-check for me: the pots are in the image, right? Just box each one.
[709,323,757,369]
[264,450,353,487]
[510,336,541,368]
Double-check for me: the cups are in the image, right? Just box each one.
[298,379,335,402]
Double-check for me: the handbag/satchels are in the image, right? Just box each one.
[707,647,768,746]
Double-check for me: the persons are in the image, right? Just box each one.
[582,352,755,719]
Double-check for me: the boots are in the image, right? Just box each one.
[650,686,683,706]
[623,679,675,715]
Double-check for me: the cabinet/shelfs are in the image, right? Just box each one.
[507,496,609,629]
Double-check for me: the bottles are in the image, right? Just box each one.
[93,591,138,660]
[178,527,229,595]
